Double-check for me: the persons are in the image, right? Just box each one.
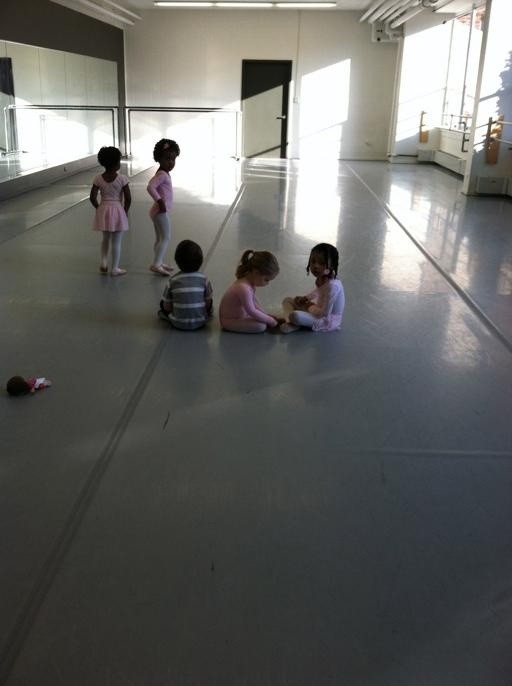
[218,249,287,334]
[88,145,132,275]
[157,238,215,332]
[146,138,180,276]
[280,240,346,334]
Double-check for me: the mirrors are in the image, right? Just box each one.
[1,38,120,185]
